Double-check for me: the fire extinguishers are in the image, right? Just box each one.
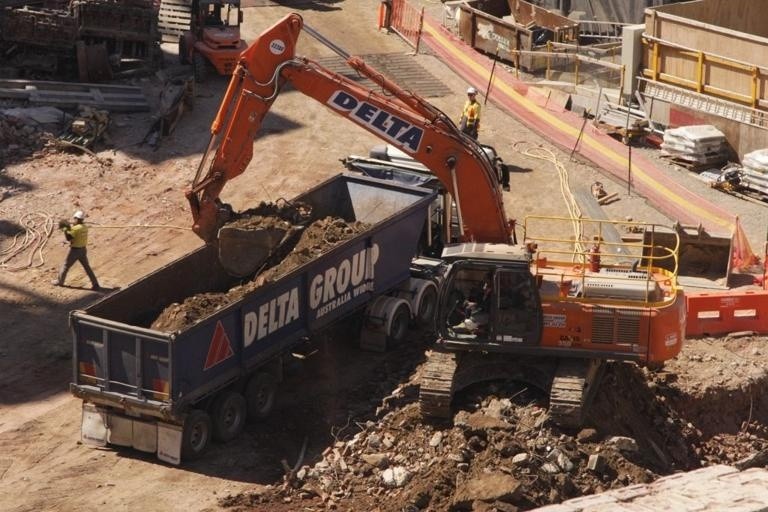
[583,244,600,271]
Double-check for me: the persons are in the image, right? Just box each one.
[51,212,100,292]
[459,87,482,139]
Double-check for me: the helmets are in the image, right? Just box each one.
[467,87,477,94]
[73,211,84,219]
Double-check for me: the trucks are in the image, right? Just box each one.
[65,139,514,470]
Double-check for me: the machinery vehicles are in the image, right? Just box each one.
[180,12,691,434]
[175,0,250,84]
